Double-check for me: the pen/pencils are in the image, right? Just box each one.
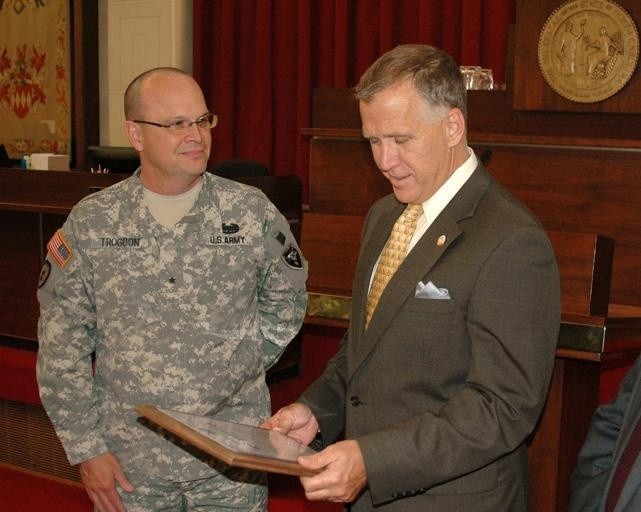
[89,164,109,175]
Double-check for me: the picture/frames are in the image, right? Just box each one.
[134,404,325,477]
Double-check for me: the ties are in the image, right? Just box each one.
[358,203,424,335]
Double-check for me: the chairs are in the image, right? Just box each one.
[86,145,139,174]
[210,159,269,184]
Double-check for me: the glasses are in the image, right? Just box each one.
[132,111,220,133]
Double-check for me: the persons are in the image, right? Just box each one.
[33,65,312,512]
[256,42,566,511]
